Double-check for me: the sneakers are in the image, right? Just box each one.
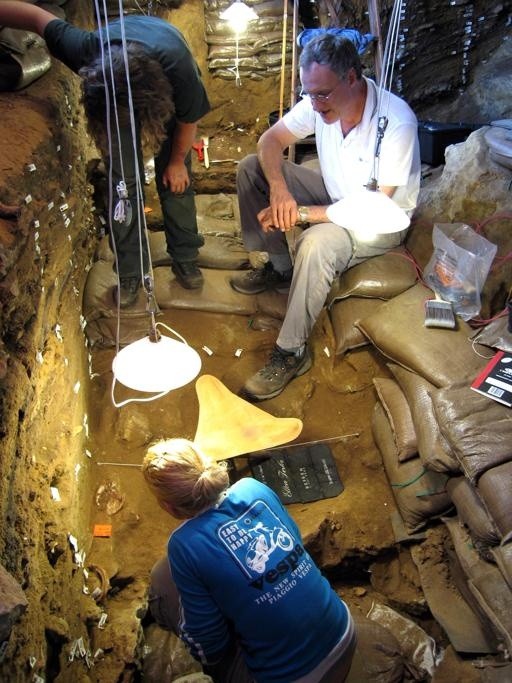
[231,270,278,294]
[114,277,141,308]
[171,261,203,288]
[245,342,313,400]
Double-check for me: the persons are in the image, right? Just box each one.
[0,0,212,309]
[229,33,421,401]
[141,436,358,683]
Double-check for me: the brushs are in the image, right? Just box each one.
[424,291,455,328]
[200,134,209,169]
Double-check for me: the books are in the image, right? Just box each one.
[470,350,512,409]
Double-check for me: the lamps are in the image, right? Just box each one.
[219,0,259,34]
[112,312,202,391]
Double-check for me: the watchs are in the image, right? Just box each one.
[298,205,309,222]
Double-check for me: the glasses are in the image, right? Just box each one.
[299,78,345,102]
